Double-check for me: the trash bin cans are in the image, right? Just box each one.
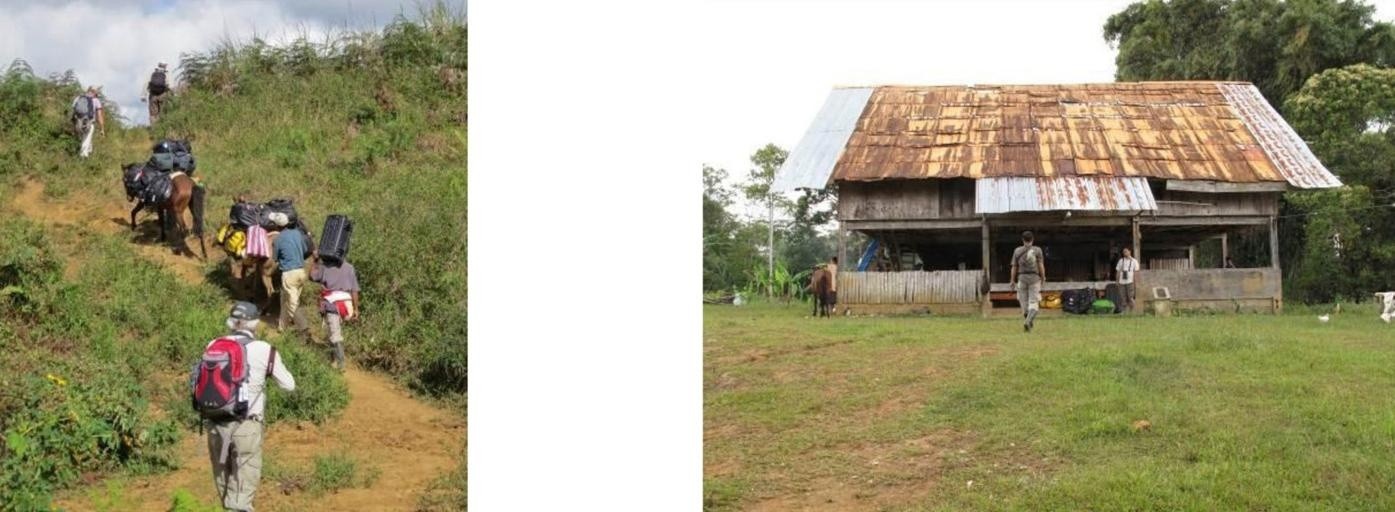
[1153,286,1171,317]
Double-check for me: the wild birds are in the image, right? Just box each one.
[1318,313,1331,323]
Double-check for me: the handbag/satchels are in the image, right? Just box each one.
[149,139,196,173]
[216,196,314,261]
[1040,284,1122,314]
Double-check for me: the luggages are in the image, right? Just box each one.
[318,214,353,263]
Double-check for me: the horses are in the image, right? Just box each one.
[231,193,280,316]
[120,161,208,259]
[811,263,838,317]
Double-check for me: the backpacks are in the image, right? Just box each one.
[74,94,95,118]
[194,334,256,419]
[150,69,169,94]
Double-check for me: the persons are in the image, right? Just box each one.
[193,302,295,512]
[828,256,840,314]
[141,61,170,124]
[1114,246,1139,315]
[71,85,106,161]
[1008,229,1046,330]
[268,211,313,342]
[1226,258,1237,268]
[310,250,361,373]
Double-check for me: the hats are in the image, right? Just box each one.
[87,86,98,92]
[158,60,169,67]
[230,302,261,320]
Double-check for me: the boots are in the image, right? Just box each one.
[329,341,346,373]
[1022,309,1038,331]
